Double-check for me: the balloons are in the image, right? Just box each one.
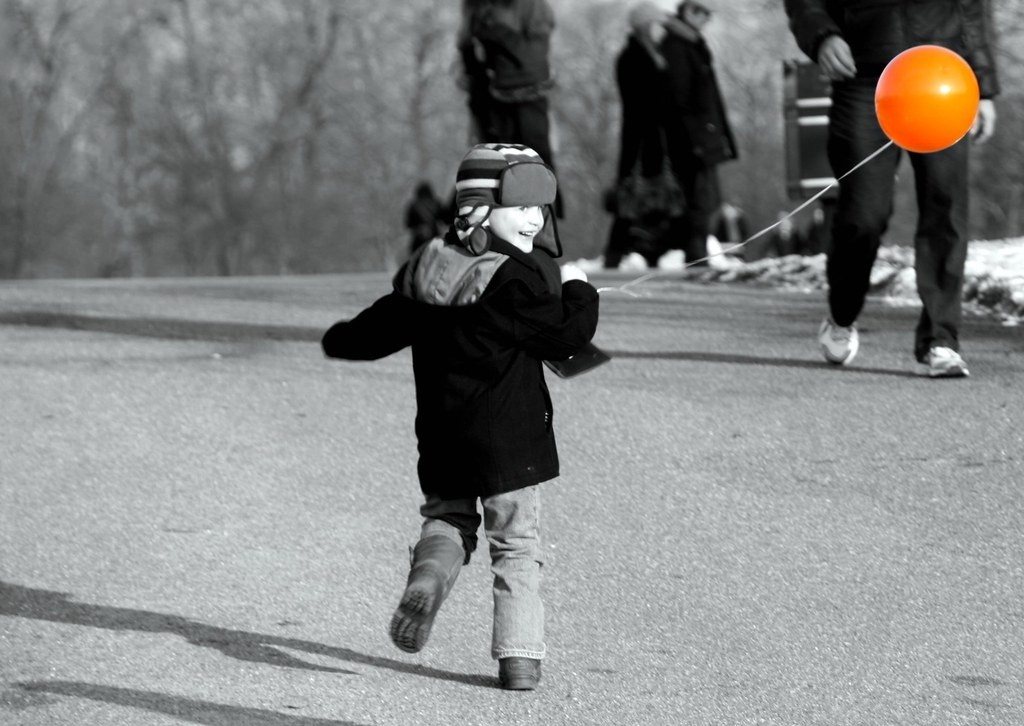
[874,45,979,154]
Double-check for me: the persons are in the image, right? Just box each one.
[322,144,599,691]
[786,0,1001,377]
[402,0,826,267]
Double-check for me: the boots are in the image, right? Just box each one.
[498,655,543,692]
[388,533,466,655]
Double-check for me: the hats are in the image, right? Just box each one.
[452,141,564,257]
[626,3,673,30]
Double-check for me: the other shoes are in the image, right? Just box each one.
[618,247,690,277]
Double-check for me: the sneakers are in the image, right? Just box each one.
[816,311,861,368]
[921,344,971,378]
